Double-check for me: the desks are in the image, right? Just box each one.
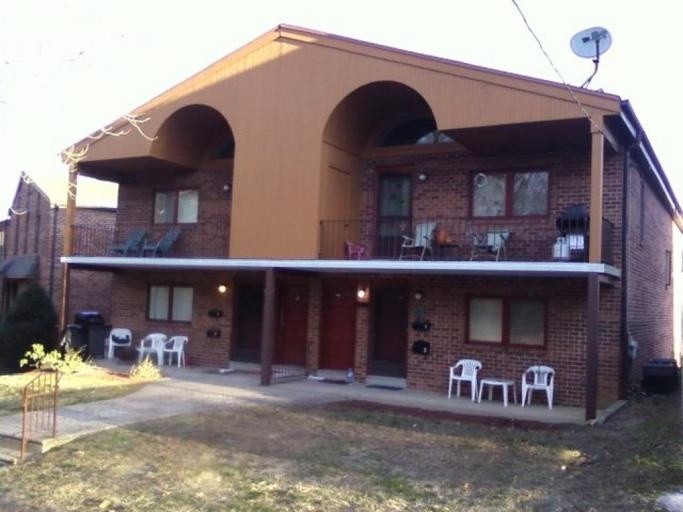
[433,234,479,262]
[476,375,517,410]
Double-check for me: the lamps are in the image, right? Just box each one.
[418,170,428,184]
[359,285,368,301]
[223,182,231,193]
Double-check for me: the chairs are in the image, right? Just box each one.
[397,222,438,260]
[104,227,145,258]
[467,229,512,261]
[519,361,556,412]
[140,228,183,259]
[446,357,481,402]
[107,326,189,370]
[344,239,369,261]
